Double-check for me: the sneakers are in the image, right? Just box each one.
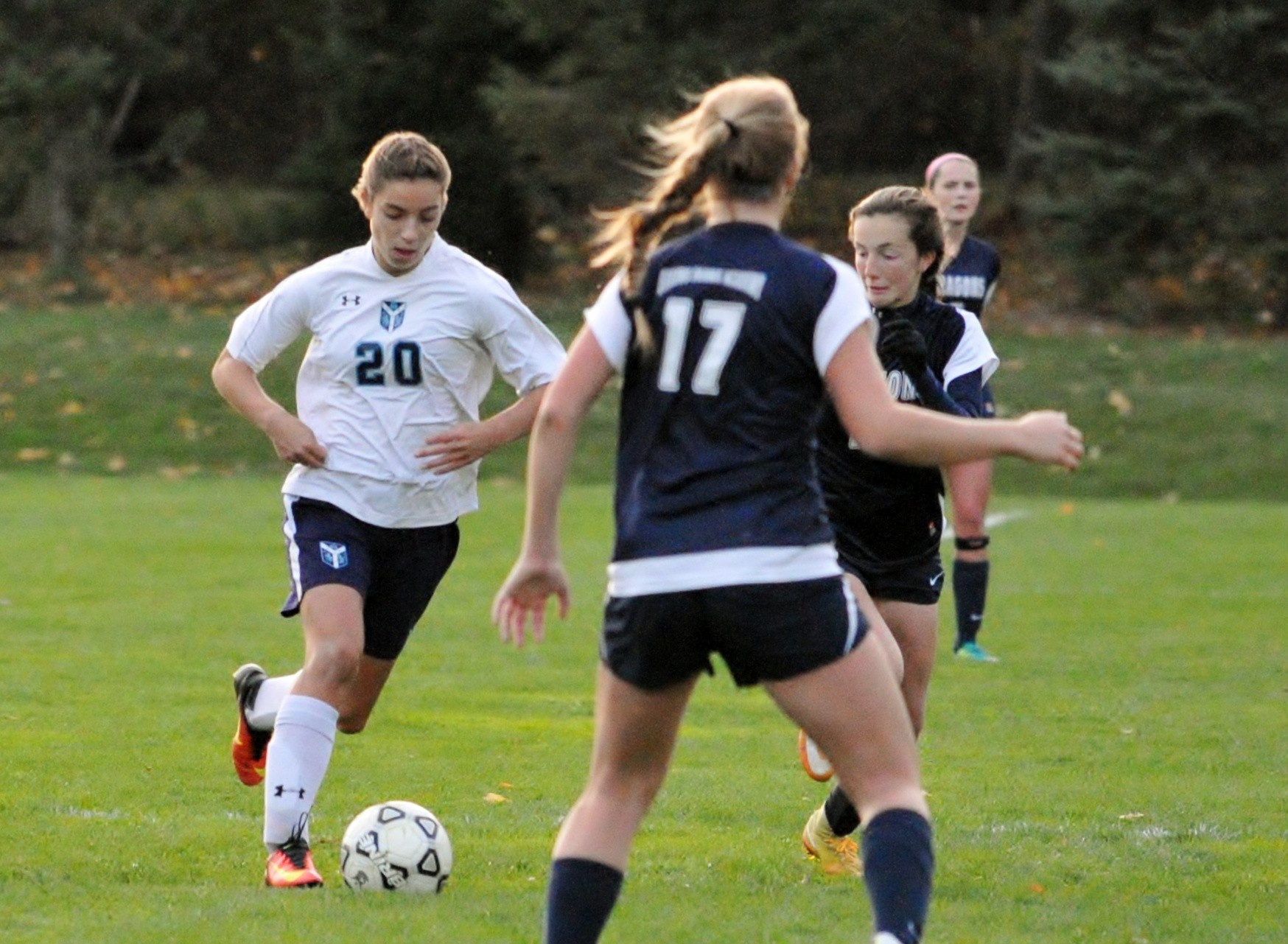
[798,728,835,782]
[952,639,998,664]
[232,663,271,787]
[264,810,324,889]
[802,801,863,878]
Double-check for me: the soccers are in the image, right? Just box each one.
[340,797,453,898]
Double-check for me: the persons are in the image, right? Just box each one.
[212,131,576,887]
[489,70,1088,943]
[798,184,1007,881]
[920,151,1003,665]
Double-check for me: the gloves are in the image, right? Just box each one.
[881,312,928,377]
